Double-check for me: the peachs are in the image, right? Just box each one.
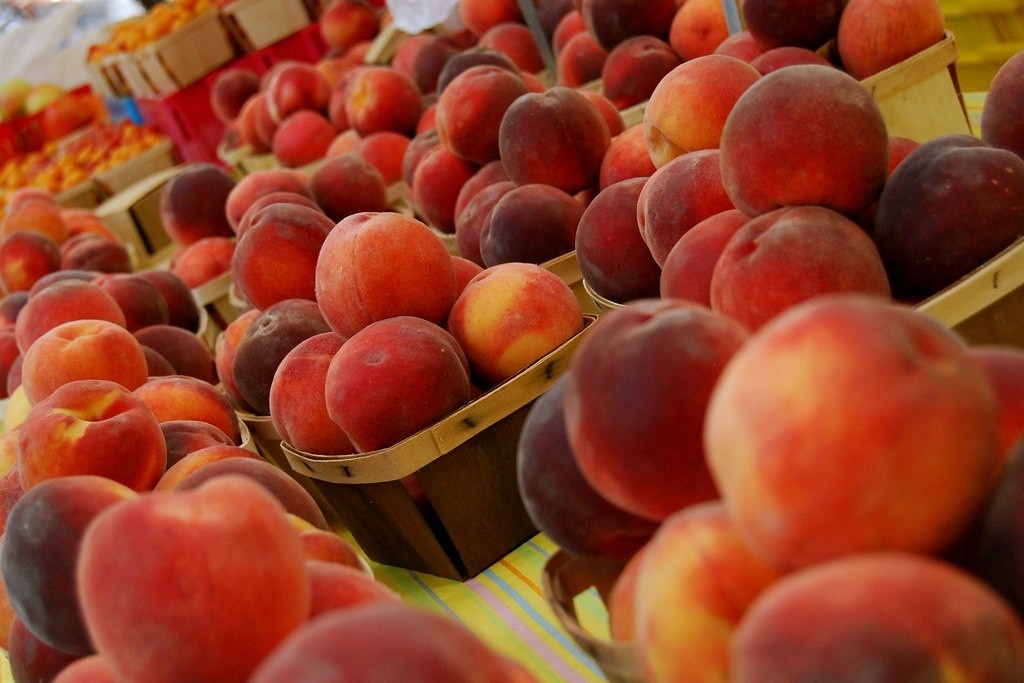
[1,1,1024,683]
